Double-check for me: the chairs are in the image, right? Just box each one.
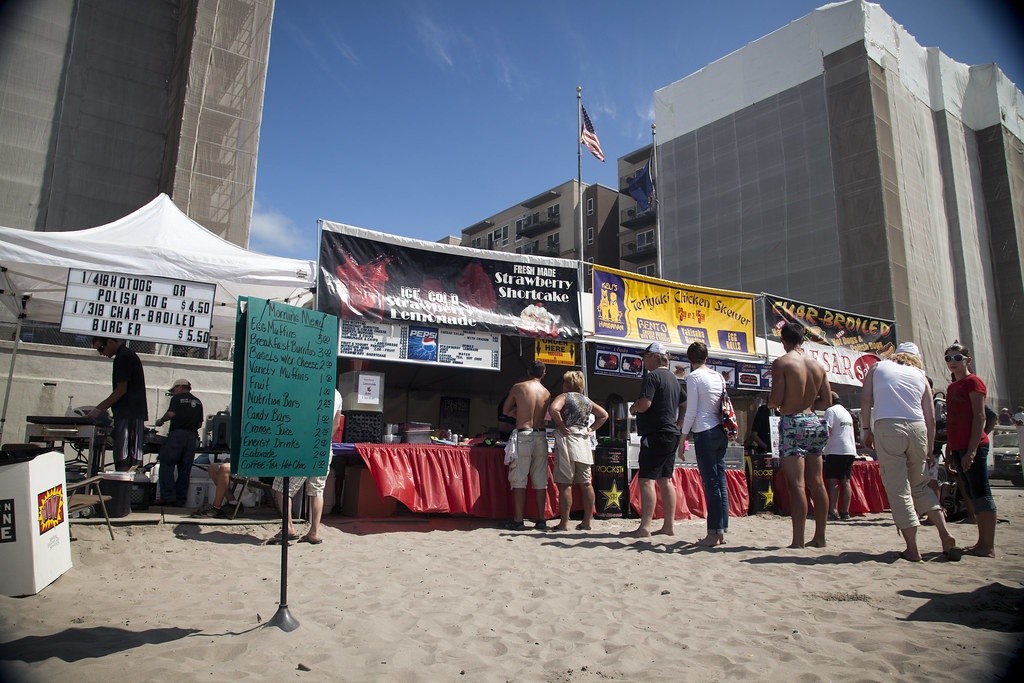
[67,475,114,542]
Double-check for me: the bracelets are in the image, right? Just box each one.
[863,427,869,430]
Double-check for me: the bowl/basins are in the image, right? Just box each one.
[382,435,401,443]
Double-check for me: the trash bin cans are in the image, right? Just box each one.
[1,445,74,597]
[96,470,136,518]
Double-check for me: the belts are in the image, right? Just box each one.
[518,427,546,432]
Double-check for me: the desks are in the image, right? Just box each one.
[629,467,749,520]
[333,442,598,523]
[773,460,891,515]
[24,423,114,519]
[197,448,231,460]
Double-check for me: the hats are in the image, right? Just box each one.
[894,341,921,359]
[168,378,191,392]
[635,341,669,356]
[831,390,840,400]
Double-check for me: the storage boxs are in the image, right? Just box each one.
[308,467,336,516]
[342,465,398,518]
[400,432,431,444]
[338,371,385,413]
[399,423,430,432]
[331,415,344,443]
[545,429,556,439]
[187,477,226,507]
[546,438,556,453]
[340,410,383,445]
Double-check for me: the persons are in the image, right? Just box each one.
[999,405,1024,426]
[860,341,961,562]
[89,337,148,471]
[915,376,943,525]
[192,463,244,518]
[678,341,730,547]
[945,340,999,558]
[623,341,687,535]
[595,393,625,437]
[271,387,343,543]
[501,360,551,530]
[768,323,833,547]
[549,371,609,531]
[750,390,857,521]
[155,379,203,506]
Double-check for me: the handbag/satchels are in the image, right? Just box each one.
[718,371,739,442]
[983,404,998,436]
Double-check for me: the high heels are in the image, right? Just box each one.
[695,532,724,547]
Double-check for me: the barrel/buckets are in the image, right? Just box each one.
[184,478,208,508]
[95,471,136,519]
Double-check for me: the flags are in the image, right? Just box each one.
[580,106,604,162]
[626,156,654,211]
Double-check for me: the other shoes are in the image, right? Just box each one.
[534,519,546,529]
[840,512,850,519]
[151,498,177,507]
[827,512,842,521]
[503,517,524,530]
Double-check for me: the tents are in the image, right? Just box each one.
[0,193,318,519]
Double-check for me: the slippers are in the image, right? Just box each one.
[268,528,300,543]
[296,533,323,545]
[893,551,922,562]
[961,545,995,558]
[575,523,592,530]
[942,546,962,561]
[551,525,568,532]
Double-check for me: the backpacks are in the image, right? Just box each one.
[932,391,949,445]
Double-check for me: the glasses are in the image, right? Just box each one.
[944,353,968,363]
[97,341,106,352]
[562,379,569,383]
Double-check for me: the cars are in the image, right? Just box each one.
[991,433,1023,486]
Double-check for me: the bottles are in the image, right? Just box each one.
[447,429,452,441]
[452,434,458,443]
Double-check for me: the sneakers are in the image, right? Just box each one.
[190,504,227,519]
[220,503,244,516]
